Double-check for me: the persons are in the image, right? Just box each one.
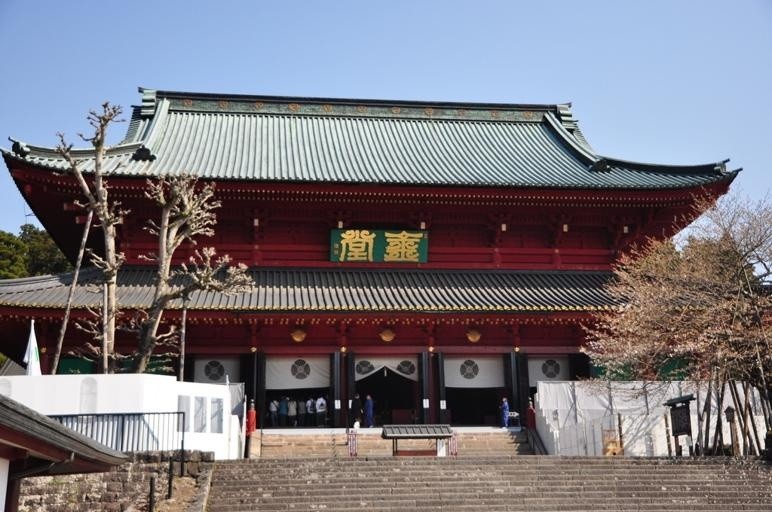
[266,391,376,427]
[497,396,511,428]
